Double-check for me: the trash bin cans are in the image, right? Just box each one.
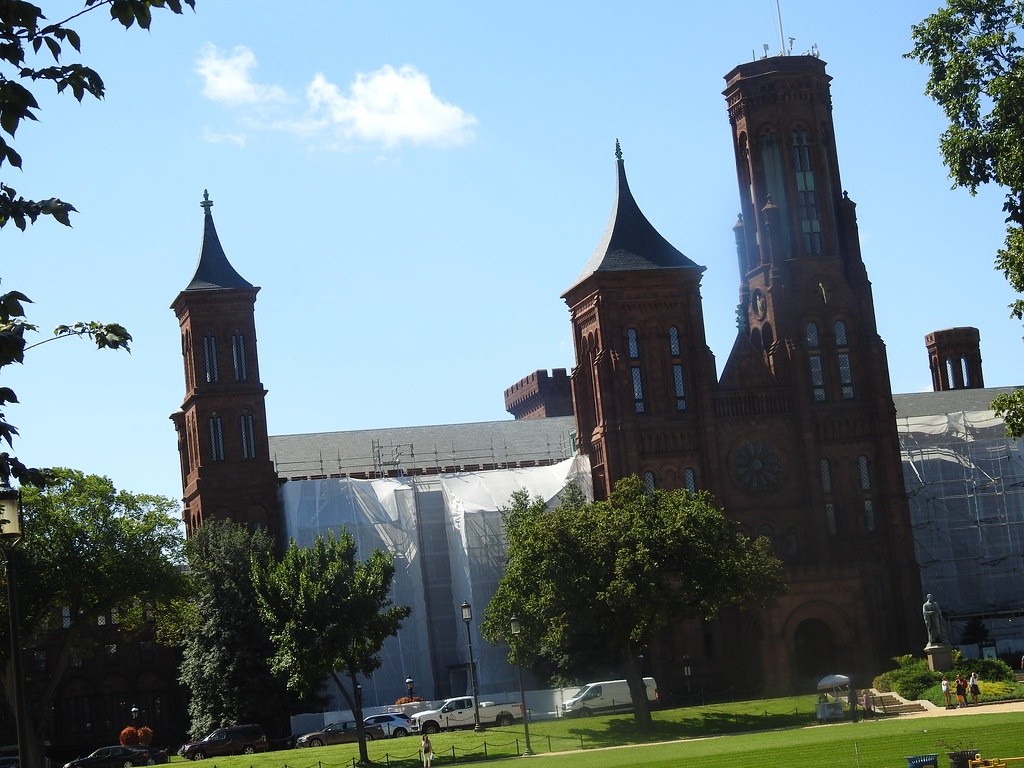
[944,749,980,768]
[905,753,940,768]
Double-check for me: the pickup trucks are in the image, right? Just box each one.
[411,696,524,735]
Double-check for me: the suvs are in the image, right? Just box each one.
[177,724,267,761]
[362,712,411,739]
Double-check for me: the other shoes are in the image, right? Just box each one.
[948,704,953,707]
[958,705,963,707]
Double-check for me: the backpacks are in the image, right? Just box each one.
[963,680,967,688]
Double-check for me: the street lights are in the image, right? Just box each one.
[461,599,484,731]
[131,704,142,766]
[683,653,693,710]
[510,613,534,755]
[405,675,414,702]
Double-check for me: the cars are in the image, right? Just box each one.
[131,745,168,765]
[296,720,384,748]
[62,746,149,768]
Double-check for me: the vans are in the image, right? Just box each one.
[560,677,659,718]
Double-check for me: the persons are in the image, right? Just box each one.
[422,734,434,768]
[942,676,954,708]
[858,690,879,723]
[956,674,968,707]
[847,686,858,723]
[969,672,981,707]
[955,681,964,708]
[923,594,946,645]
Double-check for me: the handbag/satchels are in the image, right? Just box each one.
[970,677,976,692]
[430,750,434,761]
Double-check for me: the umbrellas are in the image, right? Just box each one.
[817,674,850,700]
